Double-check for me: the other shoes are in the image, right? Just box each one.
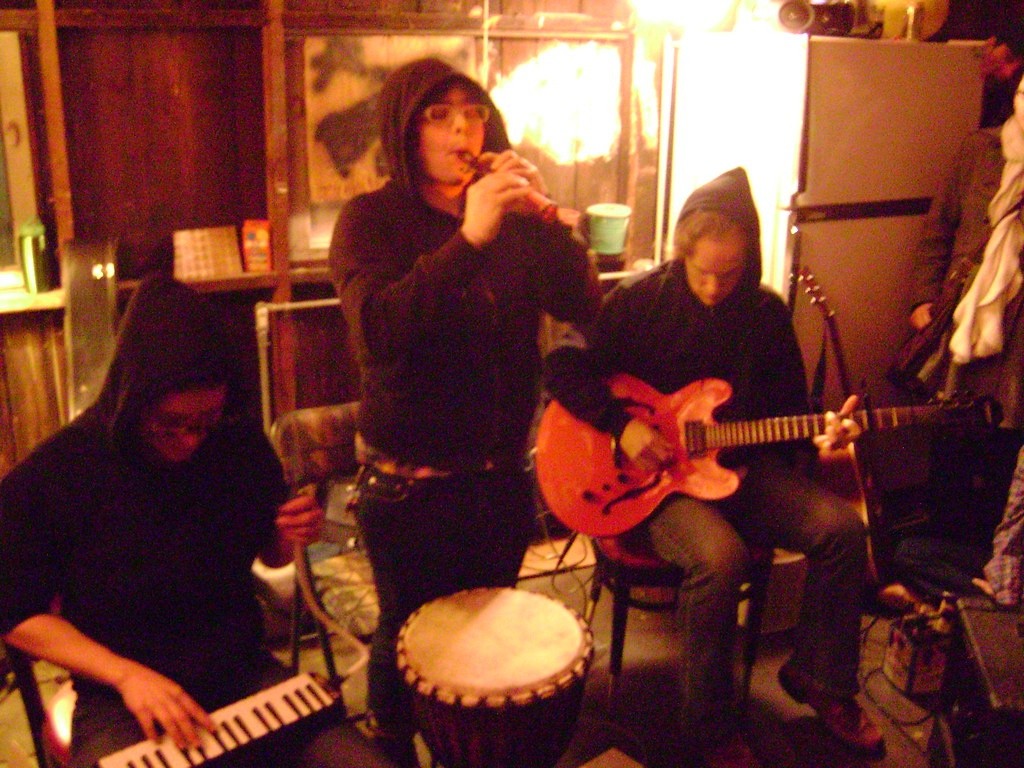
[779,659,884,754]
[690,723,760,768]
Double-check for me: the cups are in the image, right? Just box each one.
[588,199,631,256]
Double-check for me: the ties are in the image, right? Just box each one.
[373,447,537,478]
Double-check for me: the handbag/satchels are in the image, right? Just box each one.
[885,284,963,398]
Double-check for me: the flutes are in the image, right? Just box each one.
[456,149,559,224]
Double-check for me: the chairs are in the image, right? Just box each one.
[6,571,287,768]
[271,401,438,768]
[854,424,1024,760]
[538,392,777,703]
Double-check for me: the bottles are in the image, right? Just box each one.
[16,215,56,293]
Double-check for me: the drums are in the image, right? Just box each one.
[395,584,596,768]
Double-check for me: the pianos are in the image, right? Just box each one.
[70,670,347,768]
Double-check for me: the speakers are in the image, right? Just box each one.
[926,597,1024,768]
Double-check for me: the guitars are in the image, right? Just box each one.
[536,370,1005,536]
[798,266,890,582]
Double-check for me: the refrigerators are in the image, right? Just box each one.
[663,27,984,423]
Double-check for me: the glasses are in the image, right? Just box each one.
[417,104,491,123]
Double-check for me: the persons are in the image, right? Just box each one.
[985,444,1024,609]
[908,69,1024,431]
[542,169,886,768]
[329,59,601,768]
[1,279,394,768]
